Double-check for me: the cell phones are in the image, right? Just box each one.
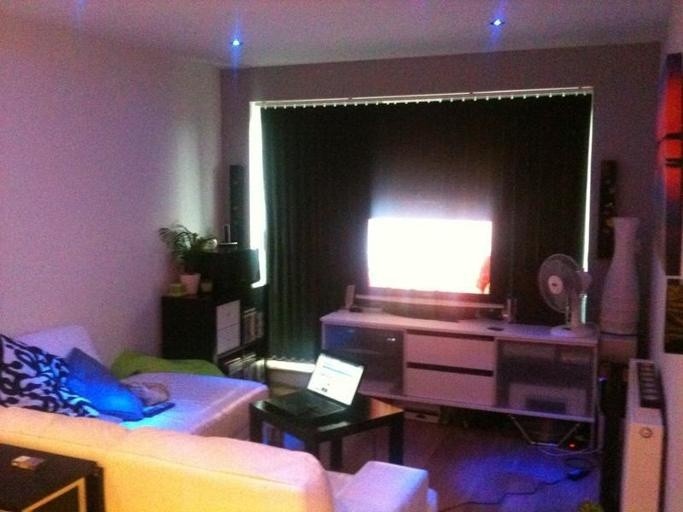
[12,455,45,471]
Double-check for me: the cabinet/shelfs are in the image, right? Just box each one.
[317,318,598,425]
[161,284,269,379]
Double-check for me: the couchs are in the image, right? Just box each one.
[0,323,441,512]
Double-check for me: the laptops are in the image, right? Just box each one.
[264,350,366,421]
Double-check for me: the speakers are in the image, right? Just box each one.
[508,298,517,324]
[344,285,355,310]
[230,165,242,244]
[597,161,617,258]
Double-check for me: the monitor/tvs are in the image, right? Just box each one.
[352,211,512,321]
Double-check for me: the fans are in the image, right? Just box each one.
[538,253,597,339]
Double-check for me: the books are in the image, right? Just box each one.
[212,298,267,385]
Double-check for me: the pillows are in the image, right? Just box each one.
[0,333,99,419]
[62,346,144,420]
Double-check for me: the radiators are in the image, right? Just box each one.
[615,357,666,512]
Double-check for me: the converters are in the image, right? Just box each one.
[567,469,584,479]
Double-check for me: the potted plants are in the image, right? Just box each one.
[157,223,216,294]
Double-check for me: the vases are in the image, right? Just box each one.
[599,215,643,335]
